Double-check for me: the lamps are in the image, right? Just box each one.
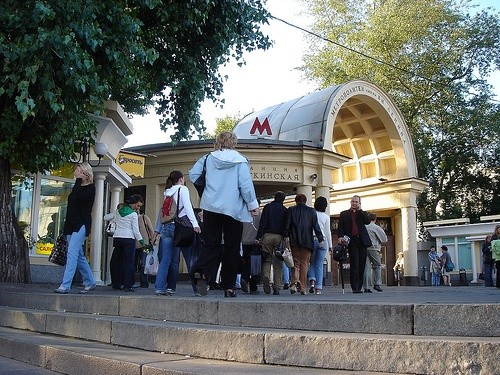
[379,178,388,182]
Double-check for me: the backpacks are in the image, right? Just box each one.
[159,194,176,224]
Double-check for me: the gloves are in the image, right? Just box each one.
[139,239,145,246]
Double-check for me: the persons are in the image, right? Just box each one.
[104,194,156,292]
[232,190,332,295]
[482,235,496,288]
[490,225,500,290]
[441,245,452,286]
[337,195,373,293]
[395,251,405,283]
[53,162,97,294]
[363,213,388,293]
[189,130,261,298]
[150,170,208,297]
[428,246,442,286]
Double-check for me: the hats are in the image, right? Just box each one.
[397,252,403,255]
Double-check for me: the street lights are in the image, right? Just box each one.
[69,137,108,168]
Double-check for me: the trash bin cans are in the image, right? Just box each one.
[323,257,328,285]
[419,265,428,286]
[459,268,467,286]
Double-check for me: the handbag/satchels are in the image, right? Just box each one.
[445,262,455,272]
[105,209,117,237]
[48,233,68,266]
[251,242,263,276]
[275,237,294,268]
[478,273,486,280]
[193,154,208,197]
[173,215,195,248]
[143,244,159,275]
[333,243,347,261]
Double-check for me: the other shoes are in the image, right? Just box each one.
[54,285,69,293]
[155,288,166,296]
[166,289,176,295]
[79,284,96,294]
[301,291,308,295]
[373,285,383,291]
[290,284,297,294]
[262,277,280,295]
[224,288,238,297]
[240,279,258,293]
[124,288,136,292]
[283,283,289,290]
[191,265,210,295]
[308,285,322,294]
